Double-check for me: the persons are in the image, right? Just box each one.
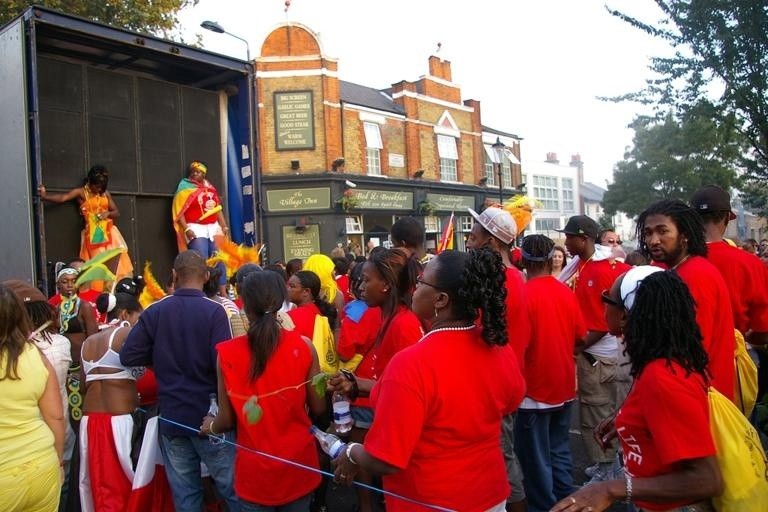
[1,161,768,511]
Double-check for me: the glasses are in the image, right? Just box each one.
[416,274,446,293]
[601,288,627,310]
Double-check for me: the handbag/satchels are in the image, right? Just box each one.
[733,326,760,420]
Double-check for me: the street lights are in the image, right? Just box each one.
[490,136,506,208]
[198,20,252,62]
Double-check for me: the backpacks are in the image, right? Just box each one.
[693,368,768,512]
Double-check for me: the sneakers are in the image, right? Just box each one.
[583,452,624,485]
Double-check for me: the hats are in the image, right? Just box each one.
[690,183,737,220]
[551,214,600,241]
[468,204,518,245]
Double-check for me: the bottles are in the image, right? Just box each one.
[332,386,354,435]
[205,392,227,445]
[308,422,349,460]
